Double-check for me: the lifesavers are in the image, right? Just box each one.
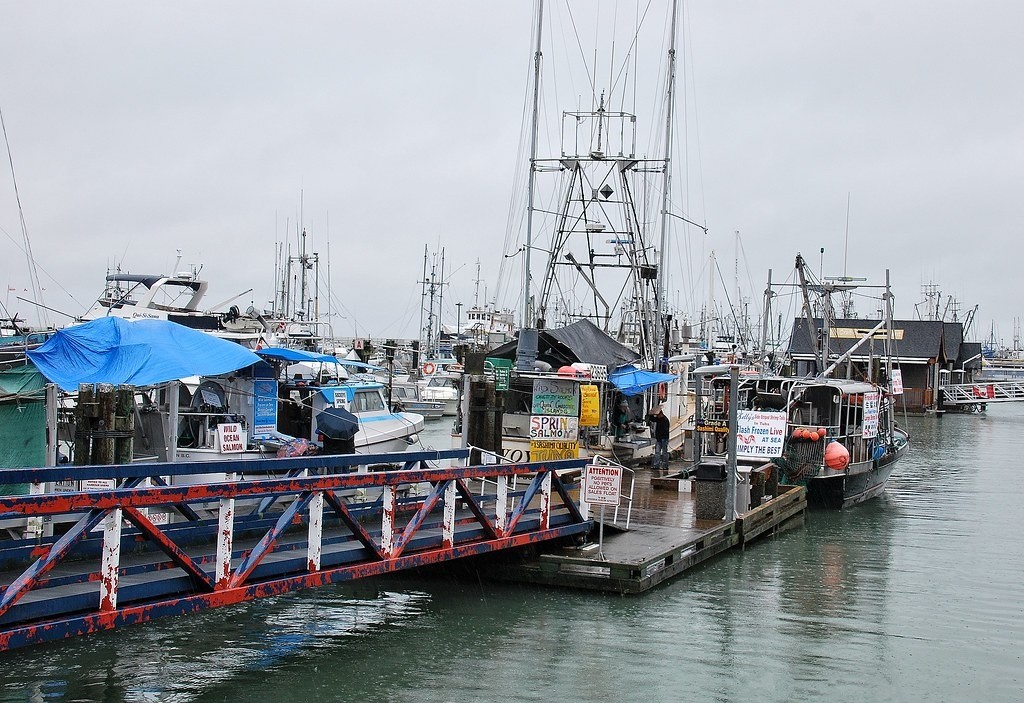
[423,362,435,374]
[659,383,666,400]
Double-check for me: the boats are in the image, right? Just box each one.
[56,0,1022,511]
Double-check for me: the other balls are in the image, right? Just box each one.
[793,427,826,441]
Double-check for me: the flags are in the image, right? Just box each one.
[279,323,286,329]
[973,384,994,399]
[256,340,264,350]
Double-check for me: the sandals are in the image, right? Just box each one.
[660,467,669,470]
[651,466,660,469]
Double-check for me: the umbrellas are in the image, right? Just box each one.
[316,407,361,442]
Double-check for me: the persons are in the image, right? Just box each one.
[610,400,630,437]
[648,410,670,471]
[322,432,358,474]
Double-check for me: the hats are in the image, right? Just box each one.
[620,400,628,406]
